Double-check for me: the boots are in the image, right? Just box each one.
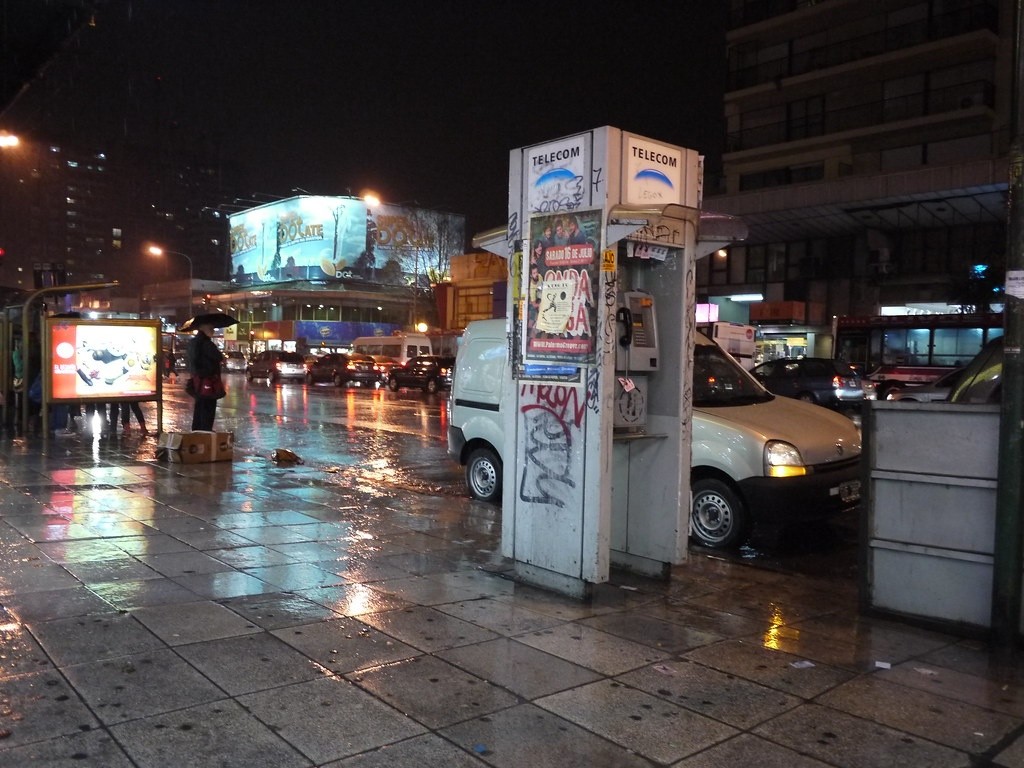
[140,421,150,435]
[122,423,131,436]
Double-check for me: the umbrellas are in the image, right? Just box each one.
[178,309,240,332]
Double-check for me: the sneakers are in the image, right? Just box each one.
[55,427,76,437]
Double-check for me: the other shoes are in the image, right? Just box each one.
[37,432,43,438]
[101,429,117,439]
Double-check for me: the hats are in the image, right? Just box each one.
[169,349,173,352]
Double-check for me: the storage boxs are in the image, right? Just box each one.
[156,430,234,464]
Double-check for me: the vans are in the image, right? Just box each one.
[162,333,194,371]
[350,335,433,368]
[447,317,859,550]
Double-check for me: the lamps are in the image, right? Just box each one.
[252,192,285,199]
[217,204,250,209]
[291,186,311,195]
[201,207,236,213]
[233,198,268,204]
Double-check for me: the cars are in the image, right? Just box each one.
[244,349,309,383]
[886,368,971,403]
[305,353,382,388]
[743,355,864,412]
[220,350,249,373]
[365,354,405,385]
[387,355,455,396]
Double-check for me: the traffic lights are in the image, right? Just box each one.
[202,296,207,309]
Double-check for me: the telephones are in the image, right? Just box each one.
[614,288,661,372]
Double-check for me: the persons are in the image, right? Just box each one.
[186,319,227,431]
[13,335,179,437]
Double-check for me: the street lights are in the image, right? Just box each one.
[149,246,193,319]
[363,192,420,334]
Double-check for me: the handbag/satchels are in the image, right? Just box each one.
[185,373,227,400]
[13,377,24,392]
